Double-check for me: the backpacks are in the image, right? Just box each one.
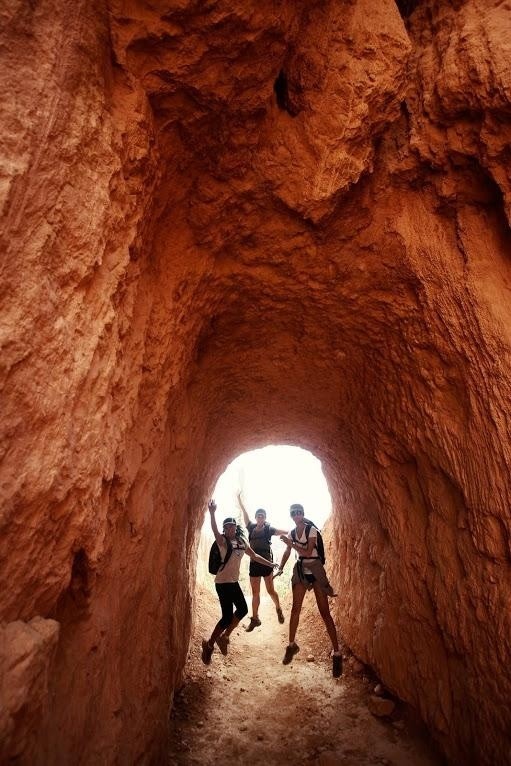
[208,534,248,575]
[291,519,325,565]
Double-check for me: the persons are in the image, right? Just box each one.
[270,501,345,678]
[199,499,280,665]
[234,487,293,634]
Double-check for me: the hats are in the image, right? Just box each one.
[255,509,266,516]
[223,518,237,526]
[291,505,304,511]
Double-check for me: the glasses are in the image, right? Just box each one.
[291,510,301,516]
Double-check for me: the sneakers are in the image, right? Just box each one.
[276,607,284,624]
[283,643,300,665]
[246,617,262,632]
[202,638,214,665]
[333,654,342,678]
[216,635,228,655]
